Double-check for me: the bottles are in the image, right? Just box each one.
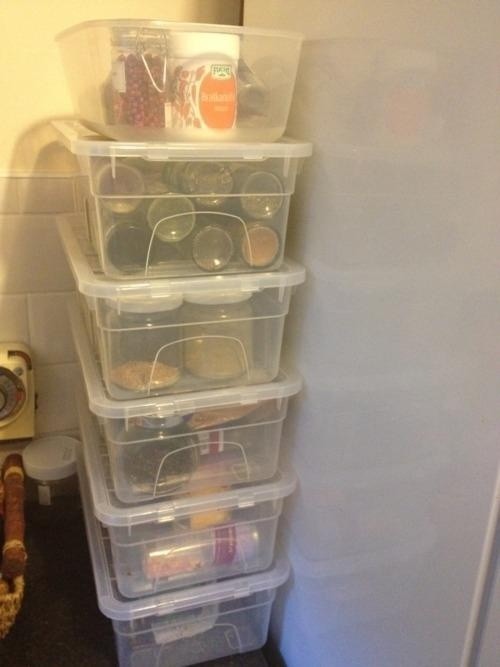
[117,413,198,495]
[139,519,260,580]
[108,27,169,131]
[184,289,255,382]
[108,293,185,392]
[20,434,84,532]
[169,32,240,141]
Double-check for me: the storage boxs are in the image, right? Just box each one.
[65,297,303,503]
[53,212,307,401]
[74,383,297,599]
[55,18,306,142]
[51,118,313,280]
[75,444,293,667]
